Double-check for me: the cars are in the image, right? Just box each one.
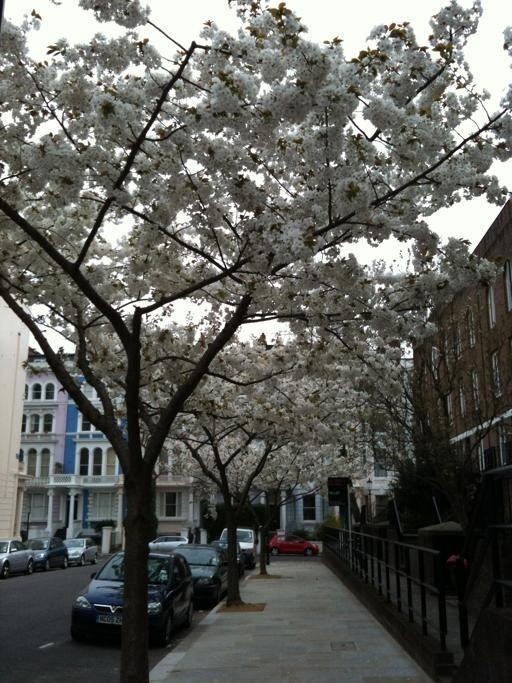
[211,539,245,579]
[148,535,189,552]
[269,533,319,556]
[169,543,229,607]
[22,536,69,570]
[70,551,194,646]
[0,537,34,579]
[61,537,99,566]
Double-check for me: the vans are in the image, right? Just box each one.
[220,527,258,569]
